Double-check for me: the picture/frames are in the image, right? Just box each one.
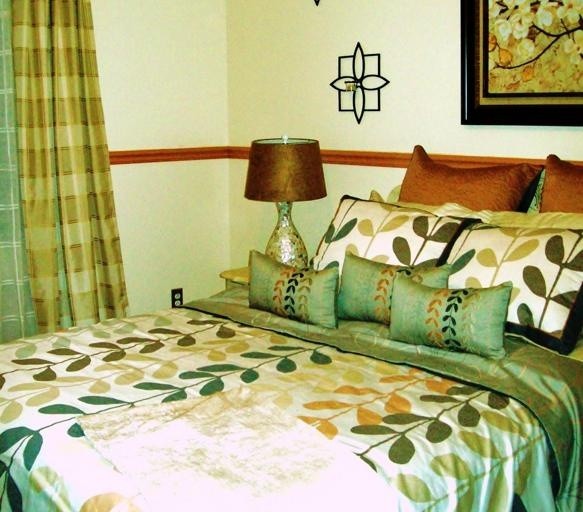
[459,0,583,128]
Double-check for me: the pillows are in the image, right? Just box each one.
[335,248,452,327]
[246,249,340,329]
[446,220,582,356]
[311,195,477,268]
[369,144,583,233]
[389,271,513,360]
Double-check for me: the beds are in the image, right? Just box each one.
[0,267,582,512]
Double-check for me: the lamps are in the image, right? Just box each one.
[243,135,327,269]
